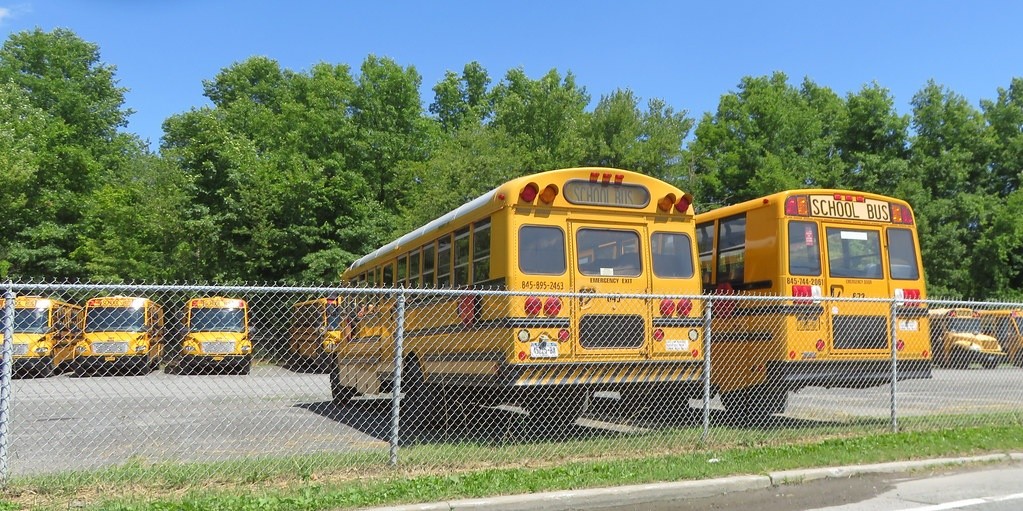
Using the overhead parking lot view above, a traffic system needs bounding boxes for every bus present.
[579,187,933,418]
[0,296,83,377]
[977,310,1023,366]
[180,297,251,373]
[928,308,1005,370]
[330,166,703,427]
[288,299,339,362]
[74,297,164,375]
